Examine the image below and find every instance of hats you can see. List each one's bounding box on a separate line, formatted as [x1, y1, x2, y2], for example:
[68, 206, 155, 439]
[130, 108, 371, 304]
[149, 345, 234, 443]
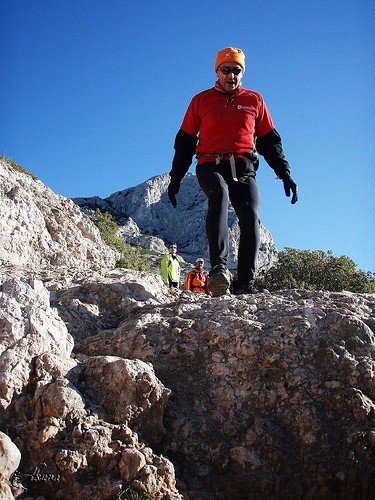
[196, 258, 204, 262]
[214, 47, 245, 73]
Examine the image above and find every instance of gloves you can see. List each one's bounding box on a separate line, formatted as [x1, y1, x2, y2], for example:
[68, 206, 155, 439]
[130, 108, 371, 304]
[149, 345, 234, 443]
[167, 176, 180, 207]
[281, 172, 298, 204]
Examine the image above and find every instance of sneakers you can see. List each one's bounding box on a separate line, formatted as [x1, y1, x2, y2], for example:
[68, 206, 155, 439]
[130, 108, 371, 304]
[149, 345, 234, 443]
[207, 265, 231, 296]
[238, 284, 271, 295]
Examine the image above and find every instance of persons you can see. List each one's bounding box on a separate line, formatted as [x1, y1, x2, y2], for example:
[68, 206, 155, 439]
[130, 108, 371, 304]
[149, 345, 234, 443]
[168, 47, 298, 297]
[161, 242, 180, 289]
[184, 258, 212, 298]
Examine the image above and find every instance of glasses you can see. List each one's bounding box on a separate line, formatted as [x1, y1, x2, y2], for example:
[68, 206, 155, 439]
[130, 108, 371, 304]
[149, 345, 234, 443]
[170, 247, 177, 248]
[218, 66, 242, 74]
